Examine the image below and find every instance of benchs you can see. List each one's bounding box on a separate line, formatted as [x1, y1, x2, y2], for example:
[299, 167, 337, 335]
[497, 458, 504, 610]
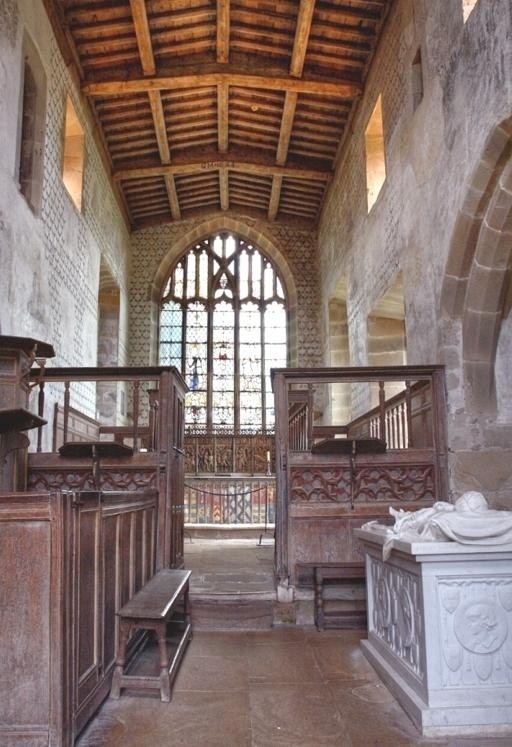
[296, 562, 368, 632]
[109, 567, 194, 703]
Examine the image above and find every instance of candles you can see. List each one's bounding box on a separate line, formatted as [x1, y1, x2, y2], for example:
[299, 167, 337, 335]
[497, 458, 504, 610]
[266, 451, 270, 463]
